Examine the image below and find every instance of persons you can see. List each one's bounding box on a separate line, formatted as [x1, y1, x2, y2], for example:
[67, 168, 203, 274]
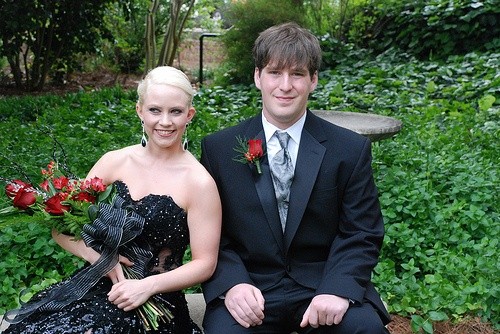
[0, 66, 222, 334]
[202, 22, 392, 334]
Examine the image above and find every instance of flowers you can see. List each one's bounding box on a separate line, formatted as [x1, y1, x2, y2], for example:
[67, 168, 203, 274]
[2, 158, 179, 333]
[231, 134, 268, 175]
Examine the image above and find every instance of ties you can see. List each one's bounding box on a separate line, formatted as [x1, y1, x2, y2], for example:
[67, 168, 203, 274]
[270, 131, 296, 232]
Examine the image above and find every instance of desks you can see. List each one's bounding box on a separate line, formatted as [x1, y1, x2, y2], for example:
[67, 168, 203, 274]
[307, 111, 402, 141]
